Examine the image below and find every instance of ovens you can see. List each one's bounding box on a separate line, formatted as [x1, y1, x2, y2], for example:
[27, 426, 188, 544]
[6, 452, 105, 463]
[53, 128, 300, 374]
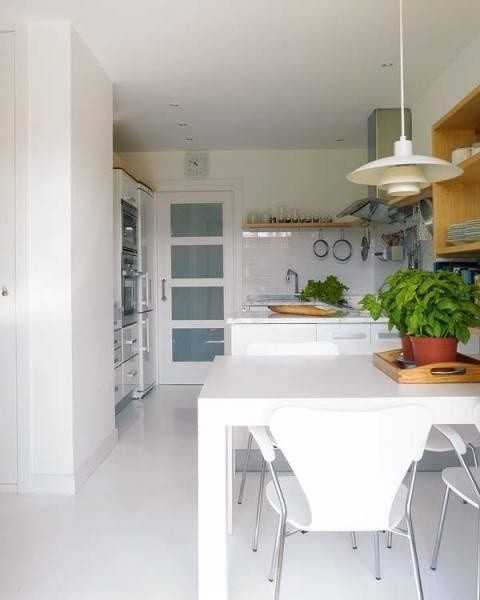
[119, 200, 141, 329]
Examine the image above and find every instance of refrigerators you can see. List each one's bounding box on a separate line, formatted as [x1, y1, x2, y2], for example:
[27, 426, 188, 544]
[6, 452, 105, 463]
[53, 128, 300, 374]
[136, 183, 156, 397]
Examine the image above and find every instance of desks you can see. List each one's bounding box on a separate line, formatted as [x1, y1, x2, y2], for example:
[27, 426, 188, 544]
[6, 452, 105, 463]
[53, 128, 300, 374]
[198, 354, 480, 600]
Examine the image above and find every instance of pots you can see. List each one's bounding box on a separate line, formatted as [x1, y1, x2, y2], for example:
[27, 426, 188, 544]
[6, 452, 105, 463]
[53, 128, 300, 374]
[313, 228, 329, 259]
[360, 228, 369, 261]
[333, 229, 352, 262]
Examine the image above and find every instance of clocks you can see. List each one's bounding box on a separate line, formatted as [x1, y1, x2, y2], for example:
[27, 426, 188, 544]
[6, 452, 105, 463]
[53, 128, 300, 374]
[183, 150, 210, 179]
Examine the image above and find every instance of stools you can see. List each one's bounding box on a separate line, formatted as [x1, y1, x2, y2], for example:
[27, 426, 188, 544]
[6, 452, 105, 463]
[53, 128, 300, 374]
[431, 405, 480, 568]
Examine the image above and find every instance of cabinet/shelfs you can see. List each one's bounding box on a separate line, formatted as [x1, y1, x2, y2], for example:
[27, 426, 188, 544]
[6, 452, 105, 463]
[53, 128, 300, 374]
[114, 322, 140, 414]
[429, 85, 479, 261]
[230, 324, 372, 353]
[362, 323, 402, 350]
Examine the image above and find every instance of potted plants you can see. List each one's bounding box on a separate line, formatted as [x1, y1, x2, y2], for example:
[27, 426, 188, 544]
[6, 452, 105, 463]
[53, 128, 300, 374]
[361, 266, 417, 361]
[398, 266, 479, 365]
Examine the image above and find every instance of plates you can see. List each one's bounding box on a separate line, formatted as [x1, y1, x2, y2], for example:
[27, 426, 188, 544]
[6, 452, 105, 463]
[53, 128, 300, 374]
[445, 219, 480, 245]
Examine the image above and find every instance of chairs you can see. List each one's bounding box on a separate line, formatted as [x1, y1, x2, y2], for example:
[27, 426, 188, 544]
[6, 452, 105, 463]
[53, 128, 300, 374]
[375, 336, 479, 547]
[242, 412, 434, 600]
[238, 341, 358, 552]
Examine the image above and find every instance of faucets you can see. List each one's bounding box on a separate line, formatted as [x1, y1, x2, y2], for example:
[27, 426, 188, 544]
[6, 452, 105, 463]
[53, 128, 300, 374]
[285, 268, 299, 294]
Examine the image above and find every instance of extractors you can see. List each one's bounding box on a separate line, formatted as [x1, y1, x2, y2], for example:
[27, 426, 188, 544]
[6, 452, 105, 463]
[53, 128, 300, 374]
[335, 109, 412, 223]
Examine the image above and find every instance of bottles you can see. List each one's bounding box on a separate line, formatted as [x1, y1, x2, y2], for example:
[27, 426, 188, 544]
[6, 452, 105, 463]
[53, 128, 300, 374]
[247, 206, 333, 224]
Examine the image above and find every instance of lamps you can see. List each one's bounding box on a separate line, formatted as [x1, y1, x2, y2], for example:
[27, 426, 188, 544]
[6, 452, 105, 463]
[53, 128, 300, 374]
[345, 1, 466, 197]
